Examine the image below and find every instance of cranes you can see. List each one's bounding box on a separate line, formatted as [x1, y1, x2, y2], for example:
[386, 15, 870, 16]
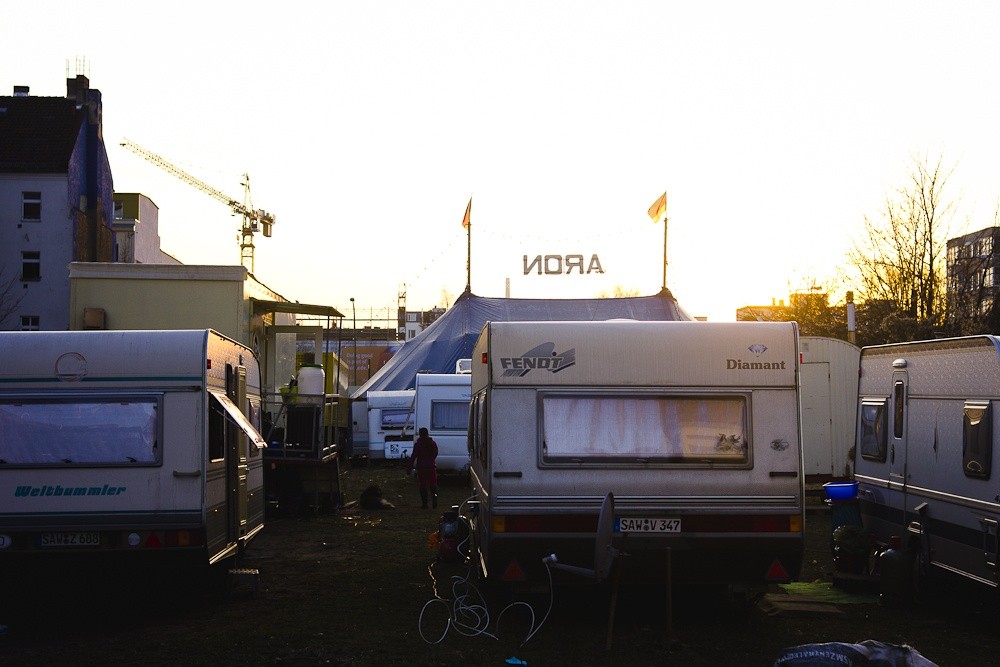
[118, 136, 276, 274]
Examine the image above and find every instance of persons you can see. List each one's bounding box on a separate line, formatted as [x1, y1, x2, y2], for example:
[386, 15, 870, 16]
[408, 428, 439, 509]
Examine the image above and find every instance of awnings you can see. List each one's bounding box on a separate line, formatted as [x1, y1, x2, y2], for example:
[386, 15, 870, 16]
[210, 390, 268, 448]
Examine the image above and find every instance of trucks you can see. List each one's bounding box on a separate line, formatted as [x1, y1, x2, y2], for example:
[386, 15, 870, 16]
[0, 329, 269, 606]
[798, 335, 861, 484]
[66, 261, 366, 515]
[415, 357, 472, 475]
[853, 331, 1000, 606]
[365, 388, 415, 465]
[466, 316, 807, 596]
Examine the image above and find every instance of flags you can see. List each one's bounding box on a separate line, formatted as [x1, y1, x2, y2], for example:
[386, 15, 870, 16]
[462, 200, 470, 229]
[647, 193, 667, 224]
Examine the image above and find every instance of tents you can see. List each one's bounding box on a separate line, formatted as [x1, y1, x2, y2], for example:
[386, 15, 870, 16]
[349, 292, 699, 399]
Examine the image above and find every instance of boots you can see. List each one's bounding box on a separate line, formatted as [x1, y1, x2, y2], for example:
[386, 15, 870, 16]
[431, 495, 438, 508]
[420, 490, 429, 509]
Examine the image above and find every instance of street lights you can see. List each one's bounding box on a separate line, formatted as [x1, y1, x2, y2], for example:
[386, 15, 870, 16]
[350, 298, 357, 386]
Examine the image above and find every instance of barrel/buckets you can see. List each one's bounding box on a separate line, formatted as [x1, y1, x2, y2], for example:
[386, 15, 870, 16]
[279, 386, 298, 405]
[298, 363, 325, 406]
